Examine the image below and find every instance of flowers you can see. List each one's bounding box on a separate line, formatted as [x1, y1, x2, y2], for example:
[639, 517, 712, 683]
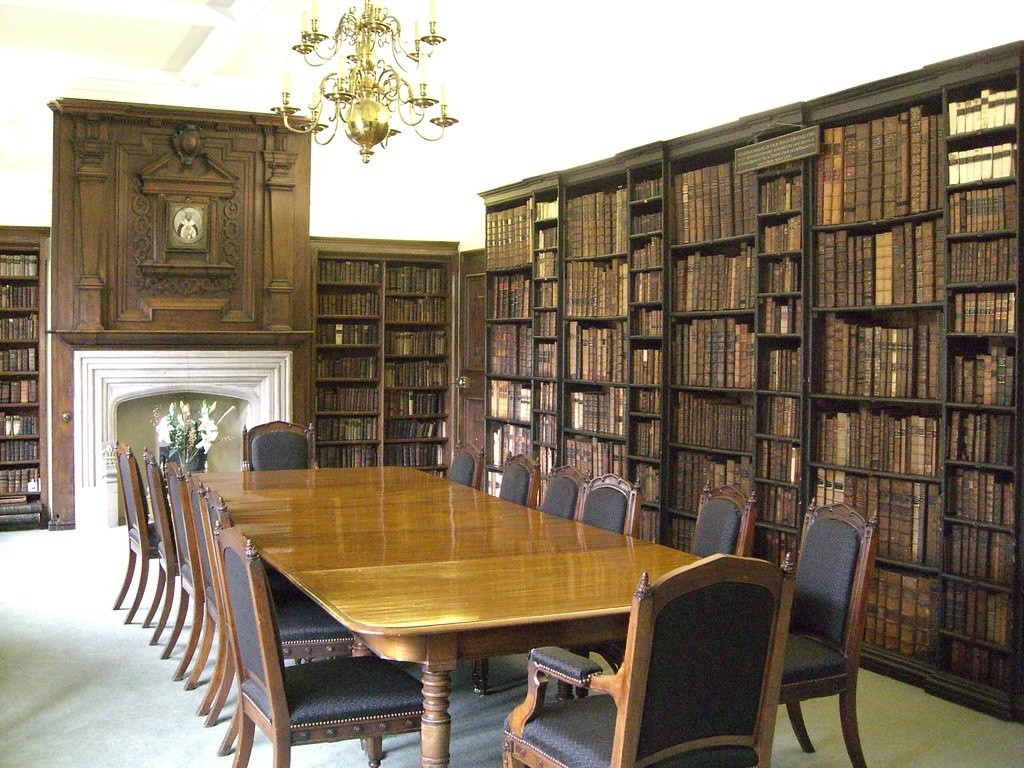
[151, 392, 236, 476]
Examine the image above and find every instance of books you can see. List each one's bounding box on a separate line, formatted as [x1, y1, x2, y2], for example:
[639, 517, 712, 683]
[300, 78, 1019, 711]
[1, 243, 57, 515]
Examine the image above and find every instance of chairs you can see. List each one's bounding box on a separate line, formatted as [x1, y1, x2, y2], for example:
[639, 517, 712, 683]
[198, 487, 352, 758]
[571, 468, 644, 537]
[688, 475, 758, 566]
[184, 471, 229, 717]
[535, 459, 583, 527]
[448, 441, 486, 494]
[108, 438, 176, 626]
[156, 458, 209, 681]
[241, 422, 319, 474]
[496, 551, 796, 767]
[209, 517, 452, 767]
[772, 495, 880, 768]
[141, 446, 191, 646]
[497, 449, 540, 511]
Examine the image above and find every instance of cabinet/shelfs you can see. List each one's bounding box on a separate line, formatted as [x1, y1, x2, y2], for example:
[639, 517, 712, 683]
[308, 236, 459, 484]
[482, 40, 1023, 725]
[0, 223, 53, 530]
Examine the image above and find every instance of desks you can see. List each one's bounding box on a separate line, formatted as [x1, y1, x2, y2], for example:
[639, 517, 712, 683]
[187, 461, 717, 766]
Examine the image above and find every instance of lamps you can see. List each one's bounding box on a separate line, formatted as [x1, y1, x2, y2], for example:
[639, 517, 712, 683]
[273, 0, 460, 164]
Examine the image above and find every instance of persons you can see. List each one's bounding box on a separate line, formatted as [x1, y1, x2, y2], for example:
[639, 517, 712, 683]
[177, 211, 199, 239]
